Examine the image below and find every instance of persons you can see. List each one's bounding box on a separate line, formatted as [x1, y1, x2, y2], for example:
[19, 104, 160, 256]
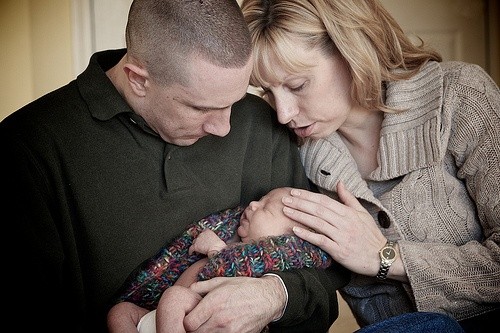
[109, 187, 311, 333]
[240, 0, 500, 333]
[0, 0, 339, 333]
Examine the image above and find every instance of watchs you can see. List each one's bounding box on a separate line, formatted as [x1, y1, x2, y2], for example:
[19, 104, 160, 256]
[376, 239, 398, 280]
